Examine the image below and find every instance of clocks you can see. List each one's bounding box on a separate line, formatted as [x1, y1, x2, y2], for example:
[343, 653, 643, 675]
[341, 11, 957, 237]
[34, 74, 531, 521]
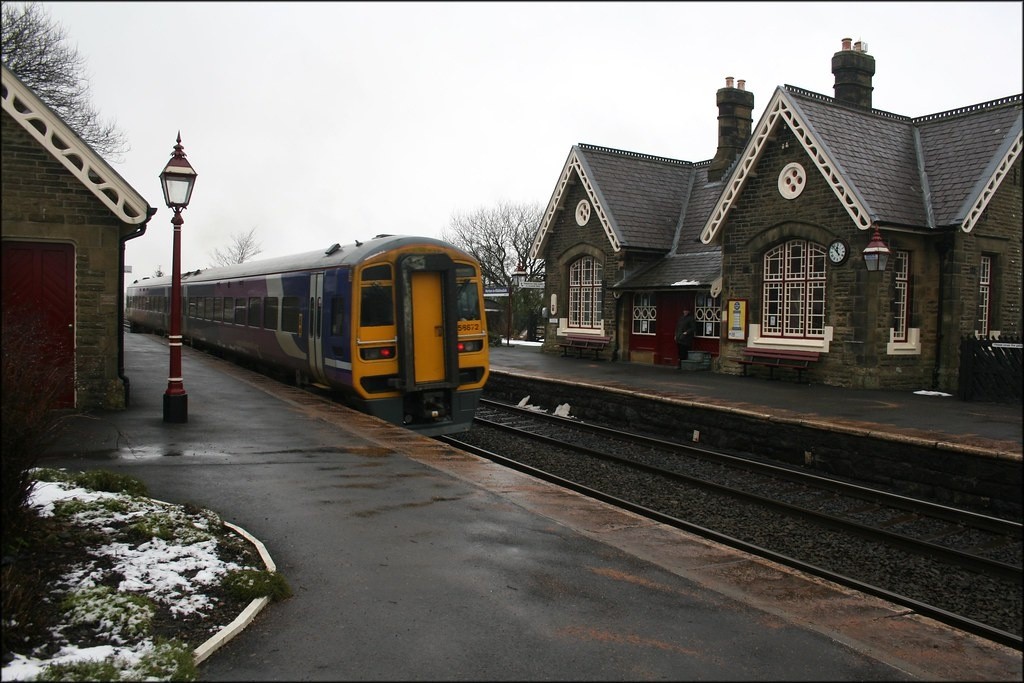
[828, 238, 849, 265]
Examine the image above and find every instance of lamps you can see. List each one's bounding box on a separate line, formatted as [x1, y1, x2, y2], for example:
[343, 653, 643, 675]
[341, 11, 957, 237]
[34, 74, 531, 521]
[863, 223, 892, 271]
[511, 263, 547, 286]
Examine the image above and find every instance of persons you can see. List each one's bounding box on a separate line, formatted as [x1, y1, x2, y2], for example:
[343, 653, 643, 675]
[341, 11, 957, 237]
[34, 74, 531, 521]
[673, 306, 697, 370]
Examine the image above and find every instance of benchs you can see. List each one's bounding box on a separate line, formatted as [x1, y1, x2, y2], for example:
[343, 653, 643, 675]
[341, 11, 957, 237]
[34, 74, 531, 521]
[734, 347, 820, 382]
[559, 333, 611, 360]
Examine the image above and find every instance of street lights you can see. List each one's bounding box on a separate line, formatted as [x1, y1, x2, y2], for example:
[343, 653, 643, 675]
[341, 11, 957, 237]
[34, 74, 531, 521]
[161, 129, 198, 426]
[506, 284, 514, 347]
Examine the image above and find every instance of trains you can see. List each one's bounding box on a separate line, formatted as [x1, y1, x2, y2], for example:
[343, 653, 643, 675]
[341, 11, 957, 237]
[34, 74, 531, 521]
[124, 232, 489, 437]
